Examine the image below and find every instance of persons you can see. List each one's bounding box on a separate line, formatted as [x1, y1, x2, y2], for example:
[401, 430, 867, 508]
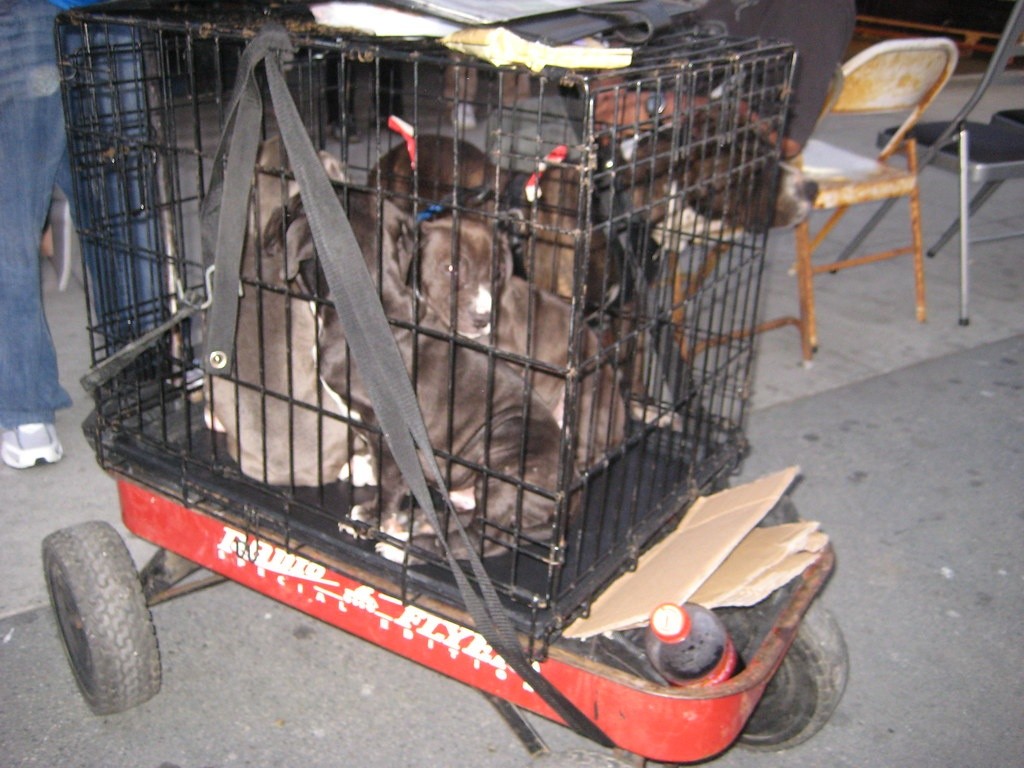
[1, 0, 202, 469]
[319, 39, 531, 142]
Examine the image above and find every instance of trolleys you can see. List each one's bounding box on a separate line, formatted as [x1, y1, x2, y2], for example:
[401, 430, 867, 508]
[34, 0, 855, 768]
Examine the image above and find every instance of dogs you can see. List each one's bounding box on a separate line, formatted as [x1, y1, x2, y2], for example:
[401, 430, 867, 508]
[202, 103, 818, 572]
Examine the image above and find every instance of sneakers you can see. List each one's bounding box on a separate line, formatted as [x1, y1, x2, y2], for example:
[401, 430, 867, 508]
[0, 422, 62, 469]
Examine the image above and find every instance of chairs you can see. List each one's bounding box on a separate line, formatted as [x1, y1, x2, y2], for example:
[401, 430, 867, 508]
[670, 38, 960, 369]
[826, 0, 1024, 327]
